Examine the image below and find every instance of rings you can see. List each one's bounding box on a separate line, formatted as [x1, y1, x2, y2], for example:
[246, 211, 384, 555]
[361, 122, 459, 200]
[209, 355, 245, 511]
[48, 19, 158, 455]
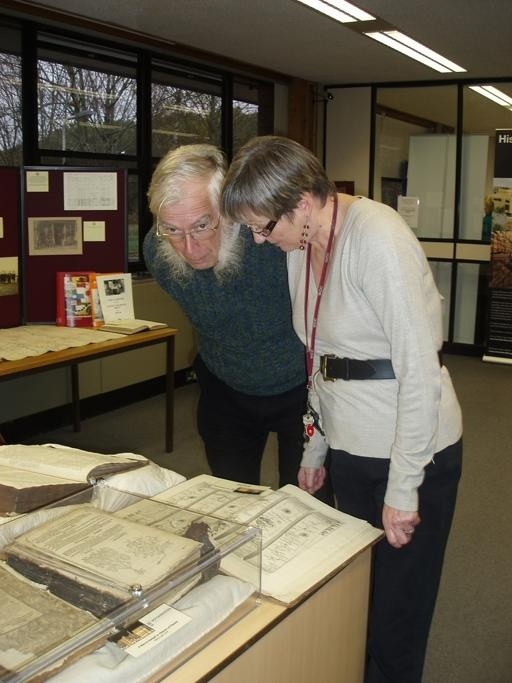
[403, 526, 415, 534]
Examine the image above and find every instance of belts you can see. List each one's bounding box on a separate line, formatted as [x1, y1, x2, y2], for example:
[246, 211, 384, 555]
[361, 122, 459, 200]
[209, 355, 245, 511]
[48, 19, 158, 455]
[320, 349, 443, 382]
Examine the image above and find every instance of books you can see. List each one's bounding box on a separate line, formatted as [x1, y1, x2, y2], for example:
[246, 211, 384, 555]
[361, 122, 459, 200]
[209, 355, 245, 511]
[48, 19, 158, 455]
[97, 317, 170, 335]
[88, 270, 125, 326]
[1, 443, 385, 681]
[96, 271, 136, 323]
[55, 270, 112, 328]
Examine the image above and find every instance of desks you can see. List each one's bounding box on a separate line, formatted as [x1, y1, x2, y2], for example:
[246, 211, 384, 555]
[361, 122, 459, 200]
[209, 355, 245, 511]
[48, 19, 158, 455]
[0, 440, 388, 681]
[1, 314, 179, 454]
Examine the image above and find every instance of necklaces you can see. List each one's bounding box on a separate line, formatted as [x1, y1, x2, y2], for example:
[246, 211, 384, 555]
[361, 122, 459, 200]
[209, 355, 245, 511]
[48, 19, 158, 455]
[299, 189, 338, 440]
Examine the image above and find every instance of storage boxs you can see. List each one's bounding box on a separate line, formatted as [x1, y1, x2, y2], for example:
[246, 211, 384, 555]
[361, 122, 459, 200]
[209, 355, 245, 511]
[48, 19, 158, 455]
[0, 482, 262, 681]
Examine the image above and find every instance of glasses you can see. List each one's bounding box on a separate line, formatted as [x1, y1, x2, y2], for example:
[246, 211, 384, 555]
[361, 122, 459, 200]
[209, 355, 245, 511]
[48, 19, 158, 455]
[156, 217, 220, 241]
[246, 215, 280, 238]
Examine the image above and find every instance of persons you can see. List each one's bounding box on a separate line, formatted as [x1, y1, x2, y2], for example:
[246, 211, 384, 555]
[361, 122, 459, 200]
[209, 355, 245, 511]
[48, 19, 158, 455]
[144, 141, 329, 499]
[221, 133, 464, 676]
